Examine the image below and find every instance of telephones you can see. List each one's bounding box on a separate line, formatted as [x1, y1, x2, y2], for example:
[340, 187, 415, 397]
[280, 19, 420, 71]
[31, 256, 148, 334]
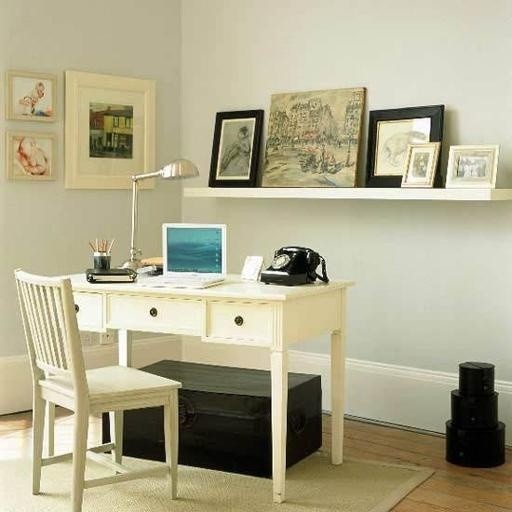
[261, 246, 330, 284]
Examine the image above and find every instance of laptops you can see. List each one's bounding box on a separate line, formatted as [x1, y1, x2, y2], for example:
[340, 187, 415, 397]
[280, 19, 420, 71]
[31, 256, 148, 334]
[137, 222, 229, 290]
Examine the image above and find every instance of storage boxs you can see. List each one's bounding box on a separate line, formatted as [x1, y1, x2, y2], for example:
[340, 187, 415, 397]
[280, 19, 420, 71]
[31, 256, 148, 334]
[100, 358, 320, 479]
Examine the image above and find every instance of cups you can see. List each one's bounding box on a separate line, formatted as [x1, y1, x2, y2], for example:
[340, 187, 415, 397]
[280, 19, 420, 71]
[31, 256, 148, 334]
[93, 251, 112, 270]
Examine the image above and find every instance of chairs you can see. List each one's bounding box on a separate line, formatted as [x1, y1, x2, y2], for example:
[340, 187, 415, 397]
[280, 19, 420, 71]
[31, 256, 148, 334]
[11, 267, 185, 512]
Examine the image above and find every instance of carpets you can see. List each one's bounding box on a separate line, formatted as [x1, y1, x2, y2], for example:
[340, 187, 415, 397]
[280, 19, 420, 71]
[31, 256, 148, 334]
[1, 435, 431, 512]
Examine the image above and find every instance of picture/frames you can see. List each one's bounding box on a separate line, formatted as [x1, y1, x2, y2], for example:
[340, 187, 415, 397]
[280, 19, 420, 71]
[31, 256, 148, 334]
[445, 143, 500, 188]
[401, 142, 441, 187]
[65, 70, 156, 191]
[6, 132, 58, 182]
[6, 70, 56, 122]
[208, 109, 263, 186]
[364, 105, 444, 187]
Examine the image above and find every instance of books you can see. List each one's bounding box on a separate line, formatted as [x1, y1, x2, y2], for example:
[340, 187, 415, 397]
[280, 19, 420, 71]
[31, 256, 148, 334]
[86, 268, 137, 283]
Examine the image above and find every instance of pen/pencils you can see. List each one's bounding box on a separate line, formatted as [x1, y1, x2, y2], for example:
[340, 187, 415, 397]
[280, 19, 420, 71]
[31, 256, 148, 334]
[87, 238, 116, 254]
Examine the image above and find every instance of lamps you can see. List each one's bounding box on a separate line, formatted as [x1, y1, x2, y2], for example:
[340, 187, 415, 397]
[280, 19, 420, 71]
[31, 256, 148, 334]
[119, 159, 200, 267]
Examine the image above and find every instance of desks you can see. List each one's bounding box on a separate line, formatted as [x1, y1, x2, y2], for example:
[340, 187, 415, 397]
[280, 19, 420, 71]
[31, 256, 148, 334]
[43, 273, 348, 503]
